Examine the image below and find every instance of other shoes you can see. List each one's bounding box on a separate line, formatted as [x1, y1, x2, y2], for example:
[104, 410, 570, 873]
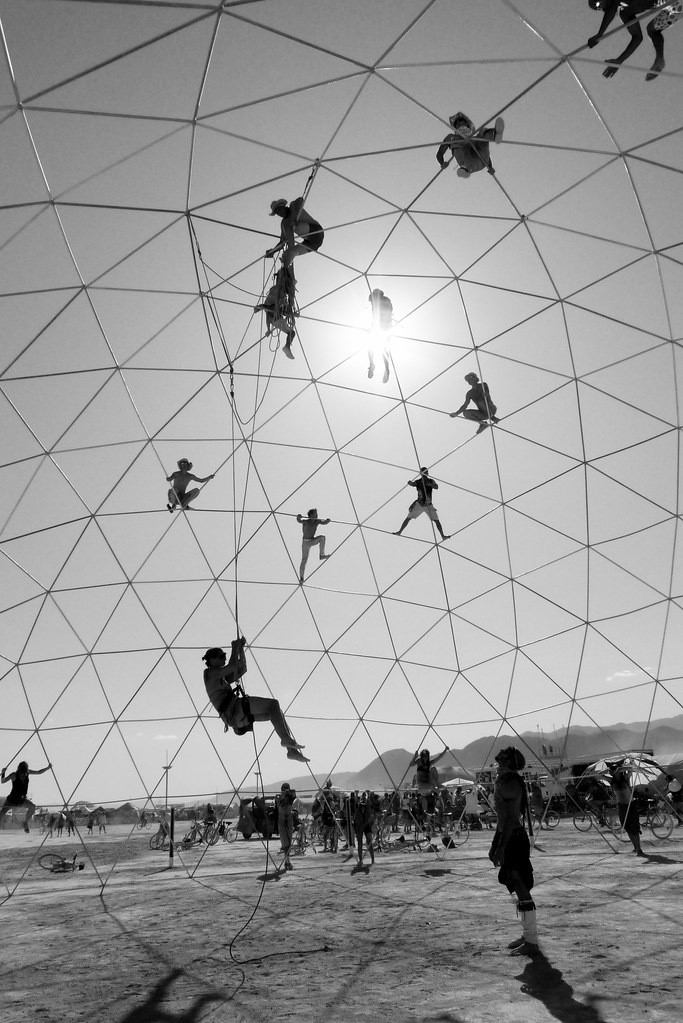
[22, 822, 30, 834]
[281, 739, 311, 763]
[382, 369, 390, 384]
[368, 364, 375, 378]
[393, 531, 401, 535]
[320, 555, 330, 560]
[282, 347, 295, 359]
[171, 504, 194, 509]
[632, 848, 644, 855]
[508, 936, 539, 956]
[495, 117, 504, 144]
[476, 418, 501, 434]
[284, 862, 294, 870]
[300, 578, 304, 583]
[442, 535, 450, 540]
[457, 167, 470, 179]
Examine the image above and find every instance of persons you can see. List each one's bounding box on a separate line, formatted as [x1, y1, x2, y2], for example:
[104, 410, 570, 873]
[449, 370, 497, 434]
[586, 0, 683, 82]
[291, 773, 555, 854]
[391, 466, 451, 540]
[201, 635, 310, 762]
[165, 456, 215, 513]
[265, 197, 325, 268]
[296, 507, 330, 584]
[410, 746, 452, 816]
[218, 820, 226, 842]
[139, 810, 147, 828]
[264, 266, 297, 359]
[488, 745, 539, 957]
[367, 287, 393, 384]
[38, 810, 107, 837]
[435, 110, 504, 178]
[354, 789, 376, 869]
[0, 761, 53, 833]
[597, 763, 644, 856]
[202, 803, 217, 828]
[275, 782, 296, 871]
[155, 815, 169, 848]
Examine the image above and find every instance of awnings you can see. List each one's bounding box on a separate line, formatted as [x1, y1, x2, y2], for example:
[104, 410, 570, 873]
[440, 777, 487, 806]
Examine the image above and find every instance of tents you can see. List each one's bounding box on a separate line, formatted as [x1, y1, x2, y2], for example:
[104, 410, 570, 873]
[292, 797, 316, 813]
[312, 785, 350, 801]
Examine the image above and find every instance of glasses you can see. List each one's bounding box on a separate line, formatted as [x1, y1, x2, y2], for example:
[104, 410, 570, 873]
[512, 746, 520, 768]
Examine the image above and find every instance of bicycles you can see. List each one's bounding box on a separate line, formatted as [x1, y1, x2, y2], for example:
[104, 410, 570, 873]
[36, 789, 683, 874]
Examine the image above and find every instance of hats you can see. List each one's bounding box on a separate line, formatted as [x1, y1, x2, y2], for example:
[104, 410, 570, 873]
[268, 199, 288, 216]
[177, 458, 194, 471]
[449, 112, 474, 131]
[465, 372, 479, 382]
[369, 287, 384, 301]
